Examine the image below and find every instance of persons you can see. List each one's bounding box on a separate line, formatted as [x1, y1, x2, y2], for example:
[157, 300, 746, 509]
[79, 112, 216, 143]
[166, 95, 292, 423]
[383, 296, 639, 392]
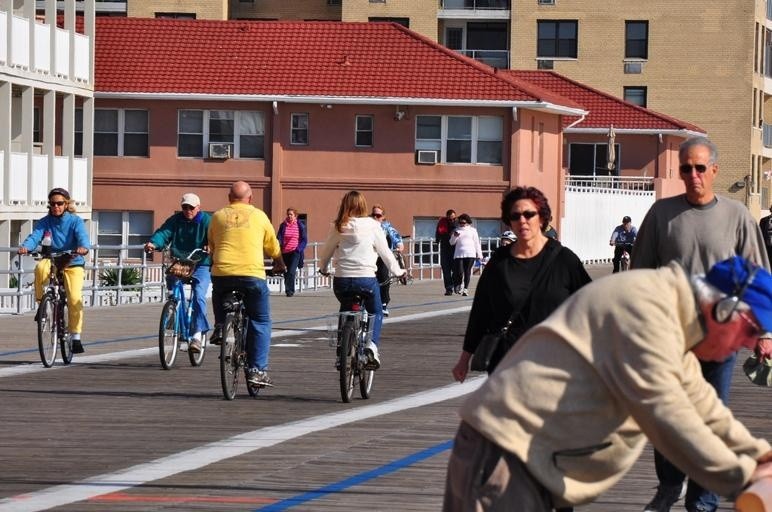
[208, 181, 282, 386]
[372, 204, 405, 314]
[452, 187, 592, 384]
[632, 138, 770, 511]
[759, 206, 772, 268]
[442, 258, 771, 512]
[320, 190, 407, 371]
[450, 214, 483, 296]
[435, 209, 463, 295]
[501, 231, 517, 247]
[276, 208, 308, 297]
[144, 193, 212, 353]
[545, 216, 558, 241]
[610, 216, 638, 274]
[18, 188, 89, 353]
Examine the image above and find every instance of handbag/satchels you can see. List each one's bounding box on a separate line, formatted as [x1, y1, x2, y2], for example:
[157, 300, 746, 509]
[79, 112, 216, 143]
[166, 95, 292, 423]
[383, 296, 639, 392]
[470, 333, 501, 372]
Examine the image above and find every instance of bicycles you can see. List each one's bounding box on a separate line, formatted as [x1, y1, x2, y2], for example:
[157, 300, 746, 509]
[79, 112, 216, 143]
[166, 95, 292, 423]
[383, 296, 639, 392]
[610, 240, 635, 274]
[145, 243, 215, 370]
[11, 245, 90, 367]
[394, 233, 417, 284]
[214, 282, 268, 399]
[315, 265, 413, 405]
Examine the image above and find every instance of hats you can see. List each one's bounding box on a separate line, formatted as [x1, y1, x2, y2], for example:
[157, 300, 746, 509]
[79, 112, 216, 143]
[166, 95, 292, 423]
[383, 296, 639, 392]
[704, 256, 771, 333]
[180, 193, 201, 208]
[49, 188, 69, 199]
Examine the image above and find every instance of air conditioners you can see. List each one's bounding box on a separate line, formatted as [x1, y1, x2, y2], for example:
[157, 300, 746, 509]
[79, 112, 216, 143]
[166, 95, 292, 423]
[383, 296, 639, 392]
[418, 150, 437, 165]
[210, 144, 230, 159]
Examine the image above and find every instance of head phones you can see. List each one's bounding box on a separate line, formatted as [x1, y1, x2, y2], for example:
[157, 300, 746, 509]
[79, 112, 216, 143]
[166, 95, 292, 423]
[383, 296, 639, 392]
[713, 265, 761, 323]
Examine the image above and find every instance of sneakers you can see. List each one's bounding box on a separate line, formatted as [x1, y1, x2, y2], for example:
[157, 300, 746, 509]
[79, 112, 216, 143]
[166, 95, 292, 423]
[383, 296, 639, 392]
[210, 323, 224, 343]
[71, 339, 84, 353]
[364, 338, 381, 369]
[188, 338, 201, 352]
[643, 480, 688, 512]
[247, 367, 276, 387]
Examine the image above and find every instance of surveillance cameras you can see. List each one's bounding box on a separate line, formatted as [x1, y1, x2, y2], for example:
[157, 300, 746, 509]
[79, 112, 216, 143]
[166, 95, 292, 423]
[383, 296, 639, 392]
[393, 111, 404, 121]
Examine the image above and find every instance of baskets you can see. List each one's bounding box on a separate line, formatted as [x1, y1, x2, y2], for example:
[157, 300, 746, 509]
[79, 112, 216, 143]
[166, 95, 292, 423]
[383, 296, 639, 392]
[163, 254, 202, 279]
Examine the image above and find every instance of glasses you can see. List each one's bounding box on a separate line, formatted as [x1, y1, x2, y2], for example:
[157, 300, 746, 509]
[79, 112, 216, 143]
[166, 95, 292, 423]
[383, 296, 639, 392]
[508, 210, 539, 222]
[680, 162, 710, 174]
[48, 200, 66, 206]
[371, 212, 382, 218]
[181, 206, 195, 210]
